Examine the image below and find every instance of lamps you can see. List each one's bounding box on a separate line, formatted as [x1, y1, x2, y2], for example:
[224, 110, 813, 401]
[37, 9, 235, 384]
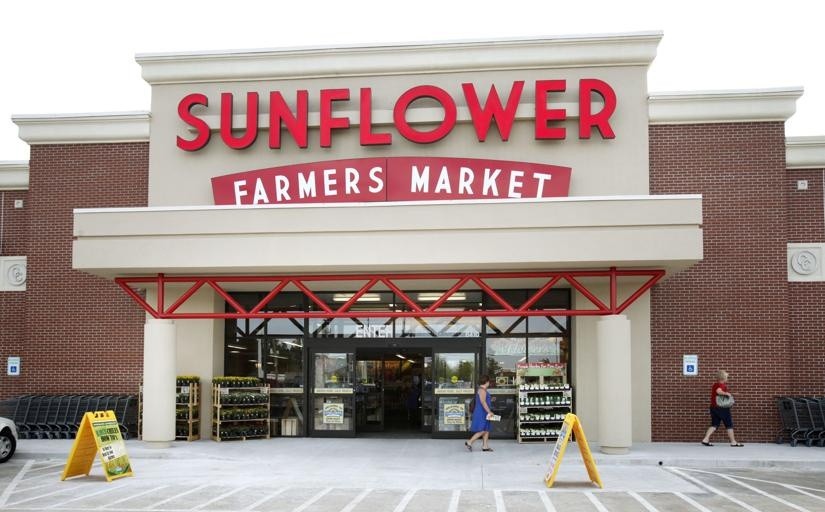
[333, 294, 381, 302]
[417, 293, 466, 301]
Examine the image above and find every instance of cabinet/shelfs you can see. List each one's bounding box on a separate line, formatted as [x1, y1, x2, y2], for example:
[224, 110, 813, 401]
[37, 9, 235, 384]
[138, 381, 202, 442]
[515, 363, 573, 444]
[211, 383, 270, 442]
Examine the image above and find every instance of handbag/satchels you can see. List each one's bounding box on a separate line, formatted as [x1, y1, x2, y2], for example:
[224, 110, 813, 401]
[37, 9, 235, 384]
[716, 395, 734, 408]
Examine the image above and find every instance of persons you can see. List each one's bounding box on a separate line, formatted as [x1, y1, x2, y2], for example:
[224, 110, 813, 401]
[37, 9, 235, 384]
[405, 378, 420, 425]
[463, 374, 495, 453]
[700, 369, 745, 448]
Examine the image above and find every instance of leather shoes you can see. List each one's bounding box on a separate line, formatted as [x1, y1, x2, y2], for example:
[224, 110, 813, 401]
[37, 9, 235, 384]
[730, 443, 745, 447]
[701, 441, 715, 446]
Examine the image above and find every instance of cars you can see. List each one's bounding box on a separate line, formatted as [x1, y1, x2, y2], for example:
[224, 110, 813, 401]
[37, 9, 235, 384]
[0, 418, 18, 463]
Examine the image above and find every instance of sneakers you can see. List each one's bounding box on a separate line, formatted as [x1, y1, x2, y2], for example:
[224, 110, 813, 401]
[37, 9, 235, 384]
[465, 441, 472, 452]
[483, 447, 493, 451]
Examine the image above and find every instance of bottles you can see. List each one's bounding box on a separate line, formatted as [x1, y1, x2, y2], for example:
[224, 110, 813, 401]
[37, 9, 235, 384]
[174, 376, 200, 436]
[211, 376, 267, 420]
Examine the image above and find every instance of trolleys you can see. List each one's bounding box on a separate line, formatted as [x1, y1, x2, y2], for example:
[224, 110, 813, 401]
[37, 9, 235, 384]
[0, 393, 138, 440]
[775, 395, 824, 446]
